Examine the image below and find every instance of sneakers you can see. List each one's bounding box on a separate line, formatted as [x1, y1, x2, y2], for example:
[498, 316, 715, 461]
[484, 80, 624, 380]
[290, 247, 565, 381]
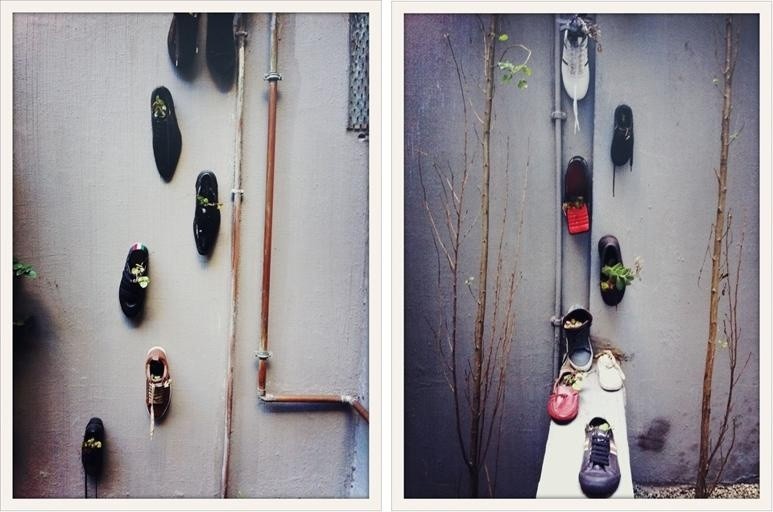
[610, 104, 634, 197]
[145, 346, 172, 440]
[560, 17, 590, 133]
[82, 417, 105, 498]
[563, 156, 589, 235]
[594, 349, 625, 392]
[578, 416, 621, 498]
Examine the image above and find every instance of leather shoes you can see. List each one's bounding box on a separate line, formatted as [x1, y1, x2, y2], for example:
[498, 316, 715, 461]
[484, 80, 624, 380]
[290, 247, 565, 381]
[193, 169, 221, 256]
[598, 235, 626, 311]
[167, 12, 201, 83]
[561, 304, 593, 373]
[119, 243, 150, 319]
[150, 85, 183, 183]
[547, 371, 579, 424]
[205, 14, 237, 95]
